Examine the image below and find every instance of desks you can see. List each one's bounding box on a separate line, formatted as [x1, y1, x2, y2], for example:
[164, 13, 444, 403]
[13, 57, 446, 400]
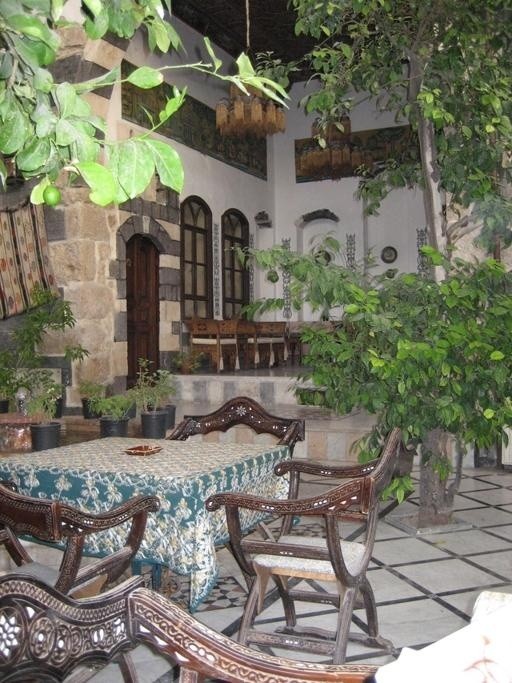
[1, 432, 293, 605]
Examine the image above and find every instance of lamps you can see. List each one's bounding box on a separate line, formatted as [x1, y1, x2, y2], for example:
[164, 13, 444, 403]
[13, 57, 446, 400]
[214, 0, 288, 139]
[296, 32, 371, 182]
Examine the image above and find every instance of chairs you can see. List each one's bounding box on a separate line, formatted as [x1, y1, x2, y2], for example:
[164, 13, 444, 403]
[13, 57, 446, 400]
[181, 313, 355, 371]
[0, 482, 163, 601]
[126, 589, 390, 681]
[206, 419, 403, 667]
[164, 394, 307, 459]
[0, 572, 150, 683]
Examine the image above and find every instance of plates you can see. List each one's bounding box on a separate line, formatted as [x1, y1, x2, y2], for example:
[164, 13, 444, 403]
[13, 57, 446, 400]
[122, 445, 163, 456]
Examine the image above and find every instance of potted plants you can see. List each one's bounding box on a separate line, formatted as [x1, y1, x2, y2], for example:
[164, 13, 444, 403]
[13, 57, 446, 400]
[1, 278, 180, 453]
